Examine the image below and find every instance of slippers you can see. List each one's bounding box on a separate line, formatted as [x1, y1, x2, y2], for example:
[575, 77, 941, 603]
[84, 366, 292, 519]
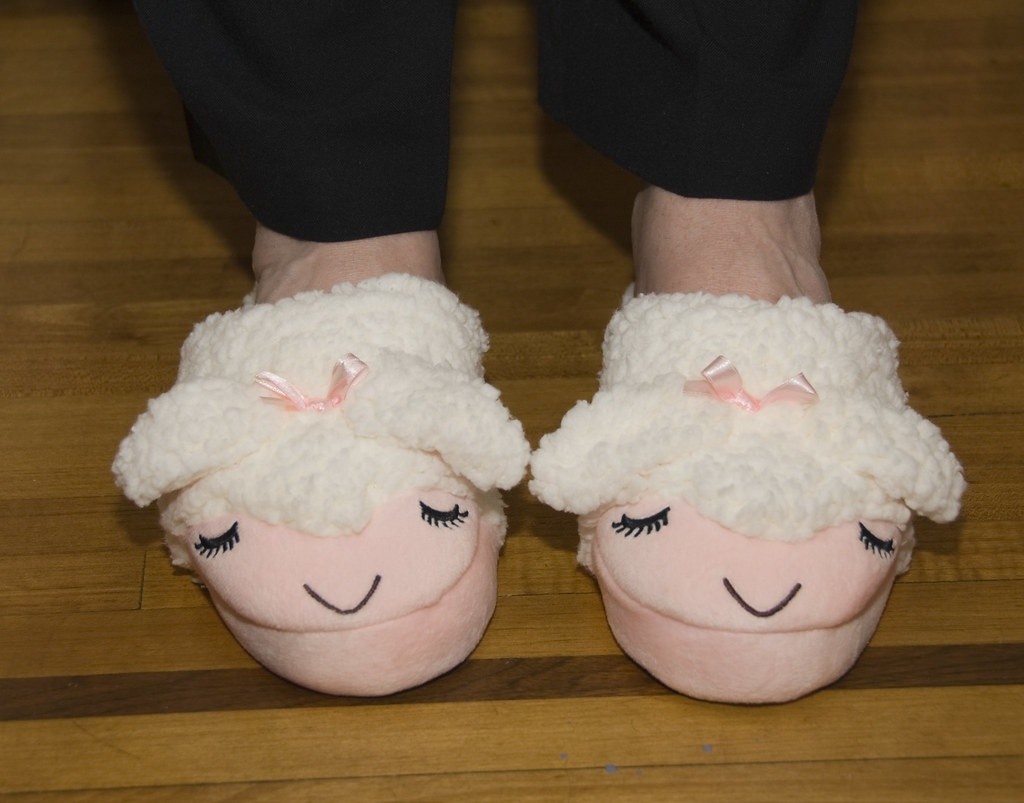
[530, 284, 967, 707]
[111, 273, 531, 696]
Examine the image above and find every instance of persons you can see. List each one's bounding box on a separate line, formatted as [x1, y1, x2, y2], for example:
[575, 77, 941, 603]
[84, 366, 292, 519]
[111, 0, 967, 704]
